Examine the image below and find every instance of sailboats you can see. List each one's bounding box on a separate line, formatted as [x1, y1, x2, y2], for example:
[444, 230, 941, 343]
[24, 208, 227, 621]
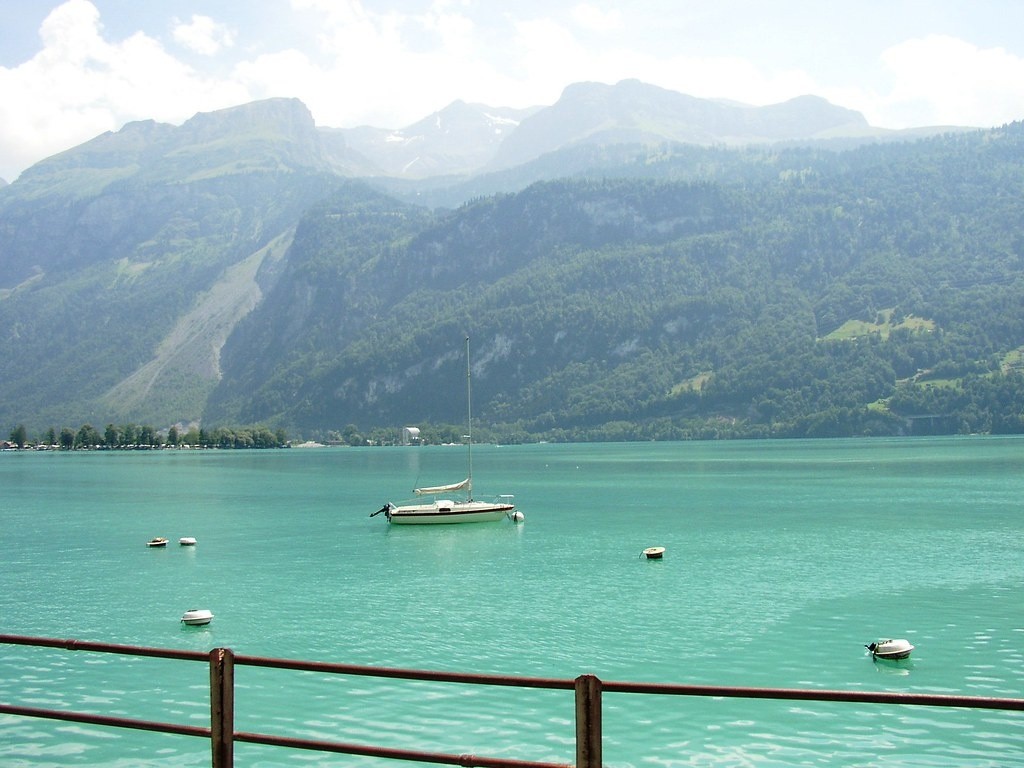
[365, 333, 518, 526]
